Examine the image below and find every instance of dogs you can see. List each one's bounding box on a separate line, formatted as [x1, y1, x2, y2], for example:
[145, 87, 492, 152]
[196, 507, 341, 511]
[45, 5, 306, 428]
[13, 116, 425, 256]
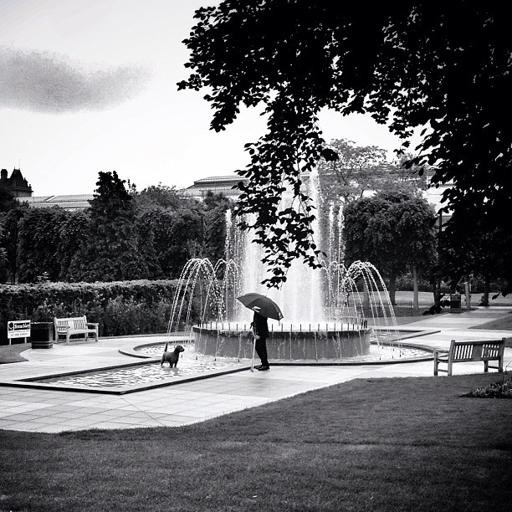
[160, 343, 184, 368]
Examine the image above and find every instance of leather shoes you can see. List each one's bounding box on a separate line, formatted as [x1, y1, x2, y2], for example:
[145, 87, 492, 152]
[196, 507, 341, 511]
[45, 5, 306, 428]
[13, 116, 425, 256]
[254, 365, 269, 371]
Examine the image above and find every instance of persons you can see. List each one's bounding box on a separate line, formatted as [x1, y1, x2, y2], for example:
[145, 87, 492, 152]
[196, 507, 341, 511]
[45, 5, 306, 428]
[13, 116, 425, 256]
[250, 310, 270, 371]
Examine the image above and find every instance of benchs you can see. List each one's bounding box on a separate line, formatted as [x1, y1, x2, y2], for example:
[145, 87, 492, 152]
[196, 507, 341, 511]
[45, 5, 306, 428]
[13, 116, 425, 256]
[54, 315, 100, 344]
[433, 337, 507, 376]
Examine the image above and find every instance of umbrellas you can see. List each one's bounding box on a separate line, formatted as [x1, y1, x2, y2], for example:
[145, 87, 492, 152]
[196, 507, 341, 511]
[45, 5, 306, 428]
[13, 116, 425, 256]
[236, 293, 284, 329]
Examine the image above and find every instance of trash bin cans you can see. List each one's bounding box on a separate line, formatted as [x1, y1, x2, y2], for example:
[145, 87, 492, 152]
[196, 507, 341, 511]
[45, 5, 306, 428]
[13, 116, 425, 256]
[451, 294, 461, 313]
[30, 322, 54, 349]
